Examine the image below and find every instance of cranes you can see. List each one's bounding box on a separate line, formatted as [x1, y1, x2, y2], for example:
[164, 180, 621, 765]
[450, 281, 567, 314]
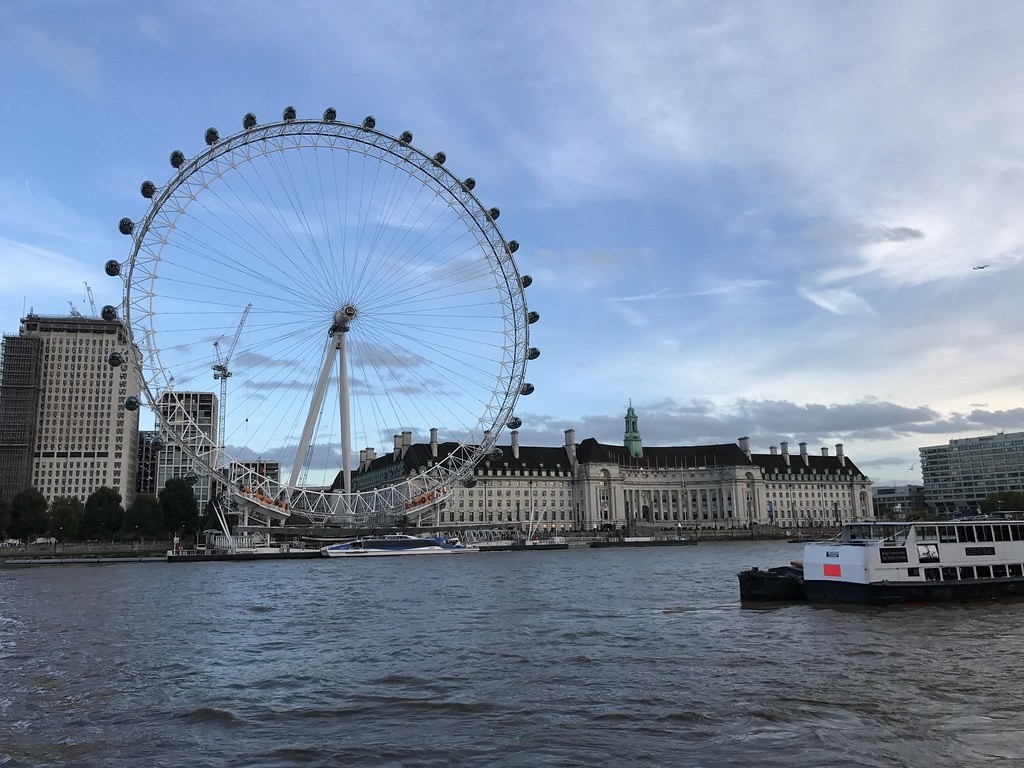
[211, 301, 254, 499]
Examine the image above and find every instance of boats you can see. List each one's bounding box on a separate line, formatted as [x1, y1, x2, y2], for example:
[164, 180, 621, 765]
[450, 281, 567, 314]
[802, 513, 1024, 602]
[736, 562, 805, 610]
[319, 535, 481, 558]
